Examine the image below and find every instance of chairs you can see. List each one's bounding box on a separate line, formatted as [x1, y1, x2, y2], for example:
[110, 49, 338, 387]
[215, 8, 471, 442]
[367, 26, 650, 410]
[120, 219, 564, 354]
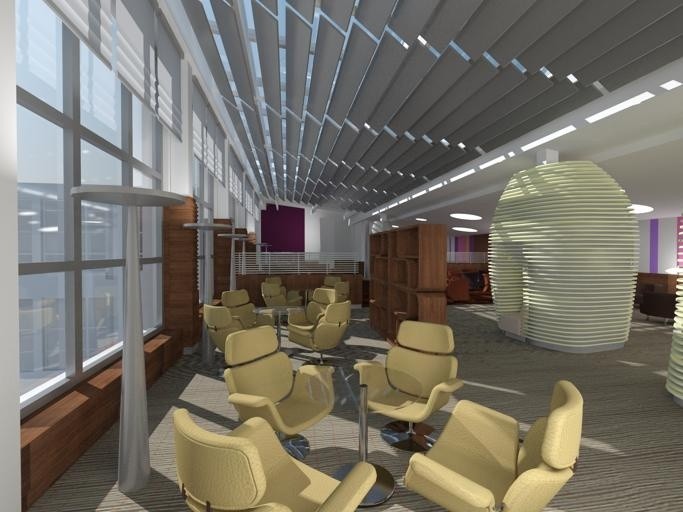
[173, 408, 378, 510]
[354, 320, 464, 452]
[405, 380, 583, 510]
[288, 301, 352, 365]
[221, 276, 352, 355]
[203, 303, 246, 377]
[222, 326, 334, 461]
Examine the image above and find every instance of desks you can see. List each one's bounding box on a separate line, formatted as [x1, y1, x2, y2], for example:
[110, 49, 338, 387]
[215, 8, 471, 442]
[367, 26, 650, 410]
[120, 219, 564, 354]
[306, 365, 423, 508]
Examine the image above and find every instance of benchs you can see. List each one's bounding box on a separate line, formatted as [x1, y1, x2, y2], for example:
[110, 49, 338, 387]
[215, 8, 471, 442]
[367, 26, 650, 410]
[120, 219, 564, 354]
[637, 290, 676, 326]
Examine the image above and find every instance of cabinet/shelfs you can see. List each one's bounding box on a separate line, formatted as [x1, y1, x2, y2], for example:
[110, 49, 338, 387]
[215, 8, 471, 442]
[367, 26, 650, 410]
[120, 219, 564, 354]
[387, 224, 450, 346]
[368, 231, 388, 338]
